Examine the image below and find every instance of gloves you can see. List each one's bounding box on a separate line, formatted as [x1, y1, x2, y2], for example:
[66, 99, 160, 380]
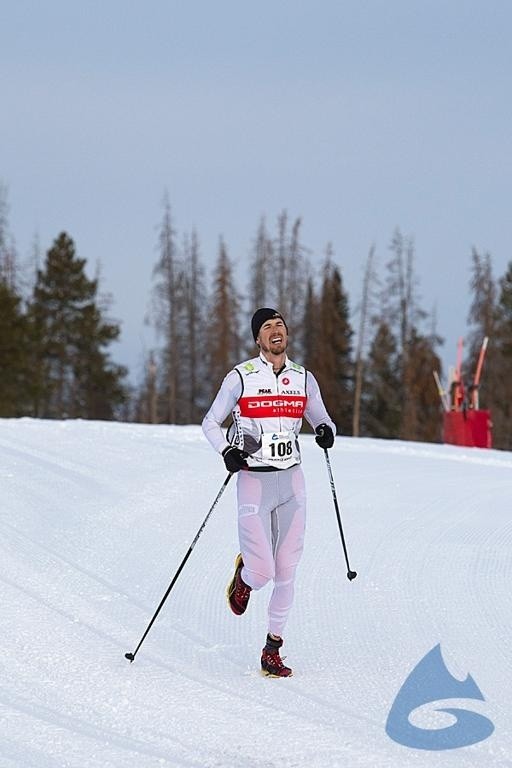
[222, 446, 250, 473]
[316, 422, 334, 449]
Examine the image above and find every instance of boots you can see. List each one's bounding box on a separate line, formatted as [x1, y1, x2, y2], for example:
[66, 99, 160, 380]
[227, 553, 252, 616]
[261, 632, 291, 679]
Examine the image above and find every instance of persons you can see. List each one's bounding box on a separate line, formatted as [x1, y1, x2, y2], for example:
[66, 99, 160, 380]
[201, 305, 337, 679]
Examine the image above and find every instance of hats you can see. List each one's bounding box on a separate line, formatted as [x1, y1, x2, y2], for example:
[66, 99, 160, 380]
[251, 307, 289, 343]
